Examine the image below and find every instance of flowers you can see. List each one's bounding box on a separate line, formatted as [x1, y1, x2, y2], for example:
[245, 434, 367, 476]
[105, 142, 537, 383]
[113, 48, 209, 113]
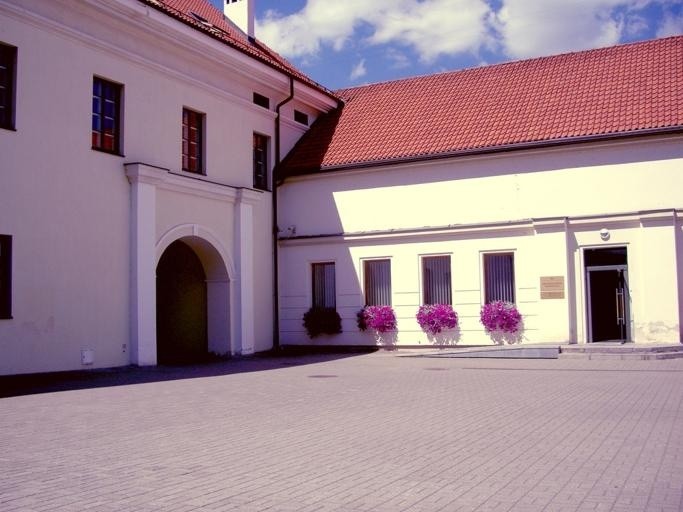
[355, 305, 398, 331]
[415, 303, 457, 335]
[303, 305, 342, 338]
[478, 300, 522, 333]
